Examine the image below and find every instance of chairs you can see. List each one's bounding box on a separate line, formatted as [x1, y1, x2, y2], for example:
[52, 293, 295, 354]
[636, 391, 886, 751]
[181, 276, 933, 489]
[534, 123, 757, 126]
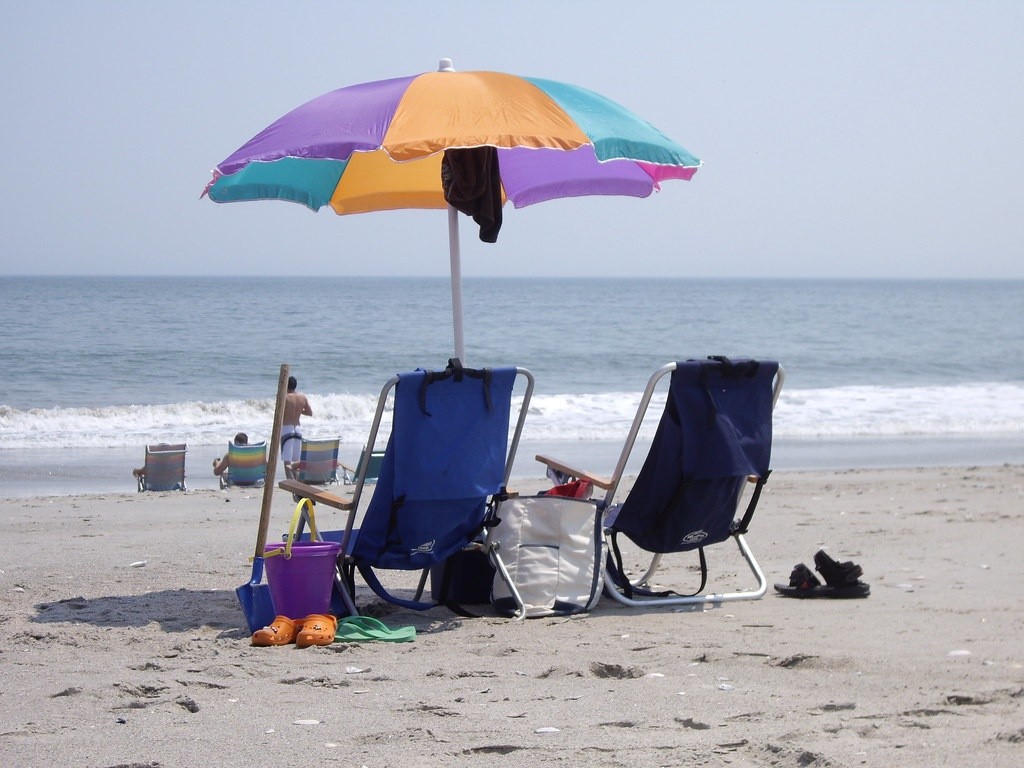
[286, 436, 341, 486]
[535, 353, 785, 608]
[278, 356, 535, 636]
[216, 440, 268, 490]
[338, 443, 385, 485]
[138, 443, 187, 492]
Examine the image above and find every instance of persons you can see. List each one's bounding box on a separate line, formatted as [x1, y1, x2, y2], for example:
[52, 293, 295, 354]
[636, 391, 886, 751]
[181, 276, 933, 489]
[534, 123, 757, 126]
[132, 442, 180, 489]
[213, 432, 248, 483]
[281, 376, 312, 480]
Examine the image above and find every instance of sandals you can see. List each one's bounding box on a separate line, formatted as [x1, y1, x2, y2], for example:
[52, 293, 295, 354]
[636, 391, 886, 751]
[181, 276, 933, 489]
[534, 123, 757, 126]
[773, 549, 874, 598]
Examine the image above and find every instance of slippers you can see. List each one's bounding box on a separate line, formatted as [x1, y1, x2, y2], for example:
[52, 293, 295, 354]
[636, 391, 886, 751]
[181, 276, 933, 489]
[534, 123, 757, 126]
[295, 612, 338, 647]
[251, 615, 306, 645]
[334, 615, 418, 643]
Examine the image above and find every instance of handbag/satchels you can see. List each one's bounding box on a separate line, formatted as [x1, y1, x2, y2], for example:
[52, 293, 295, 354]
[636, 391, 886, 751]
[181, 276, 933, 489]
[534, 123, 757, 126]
[490, 493, 613, 618]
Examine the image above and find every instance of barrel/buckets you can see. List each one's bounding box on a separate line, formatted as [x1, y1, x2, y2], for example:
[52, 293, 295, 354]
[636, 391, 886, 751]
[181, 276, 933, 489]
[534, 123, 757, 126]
[235, 498, 342, 635]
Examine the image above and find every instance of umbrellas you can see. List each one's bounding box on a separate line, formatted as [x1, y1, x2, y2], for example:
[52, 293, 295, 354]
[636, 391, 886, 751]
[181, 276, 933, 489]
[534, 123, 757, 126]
[196, 59, 707, 370]
